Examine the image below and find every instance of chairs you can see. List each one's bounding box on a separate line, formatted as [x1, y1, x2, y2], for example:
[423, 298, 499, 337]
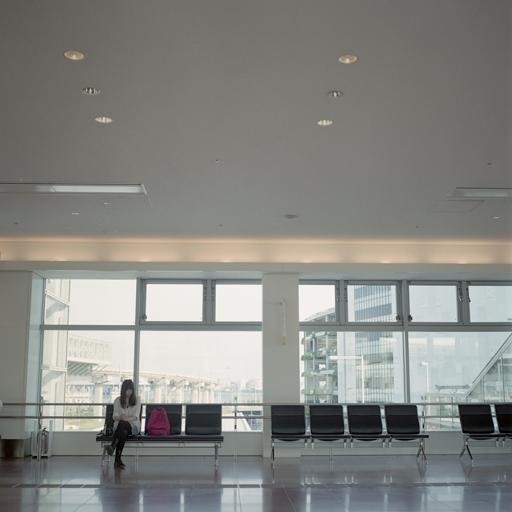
[457, 402, 511, 460]
[96, 403, 224, 462]
[270, 404, 429, 462]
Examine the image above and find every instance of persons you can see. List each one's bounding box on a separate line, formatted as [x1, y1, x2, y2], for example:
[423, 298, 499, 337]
[104, 381, 141, 469]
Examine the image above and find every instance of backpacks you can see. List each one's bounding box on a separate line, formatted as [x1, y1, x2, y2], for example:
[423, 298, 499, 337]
[146, 407, 171, 436]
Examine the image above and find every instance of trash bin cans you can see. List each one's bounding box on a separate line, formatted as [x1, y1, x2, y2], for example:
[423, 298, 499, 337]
[31, 430, 52, 458]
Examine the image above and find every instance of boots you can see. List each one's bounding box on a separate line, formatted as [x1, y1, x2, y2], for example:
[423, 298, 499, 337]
[114, 449, 126, 469]
[104, 437, 119, 456]
